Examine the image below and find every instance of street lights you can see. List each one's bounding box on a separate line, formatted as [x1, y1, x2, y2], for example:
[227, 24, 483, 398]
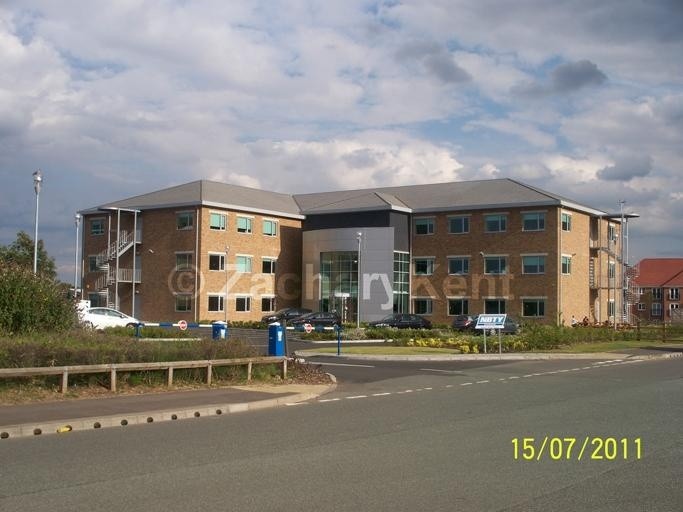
[357, 232, 362, 328]
[75, 213, 81, 298]
[225, 246, 230, 323]
[33, 170, 42, 273]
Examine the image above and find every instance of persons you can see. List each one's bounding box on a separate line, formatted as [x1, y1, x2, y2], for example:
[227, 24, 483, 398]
[571, 315, 577, 328]
[583, 316, 589, 326]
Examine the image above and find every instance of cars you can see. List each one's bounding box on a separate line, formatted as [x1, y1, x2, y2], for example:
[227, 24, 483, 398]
[77, 306, 140, 329]
[288, 310, 340, 326]
[369, 313, 432, 329]
[454, 314, 519, 335]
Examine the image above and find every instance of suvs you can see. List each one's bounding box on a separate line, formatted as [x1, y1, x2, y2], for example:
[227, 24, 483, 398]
[263, 306, 311, 323]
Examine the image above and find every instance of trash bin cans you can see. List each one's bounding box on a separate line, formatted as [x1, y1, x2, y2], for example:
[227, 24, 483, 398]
[211, 321, 227, 341]
[267, 322, 284, 355]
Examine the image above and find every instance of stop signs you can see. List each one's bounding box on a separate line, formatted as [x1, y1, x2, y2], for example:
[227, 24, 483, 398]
[179, 320, 188, 330]
[304, 324, 312, 333]
